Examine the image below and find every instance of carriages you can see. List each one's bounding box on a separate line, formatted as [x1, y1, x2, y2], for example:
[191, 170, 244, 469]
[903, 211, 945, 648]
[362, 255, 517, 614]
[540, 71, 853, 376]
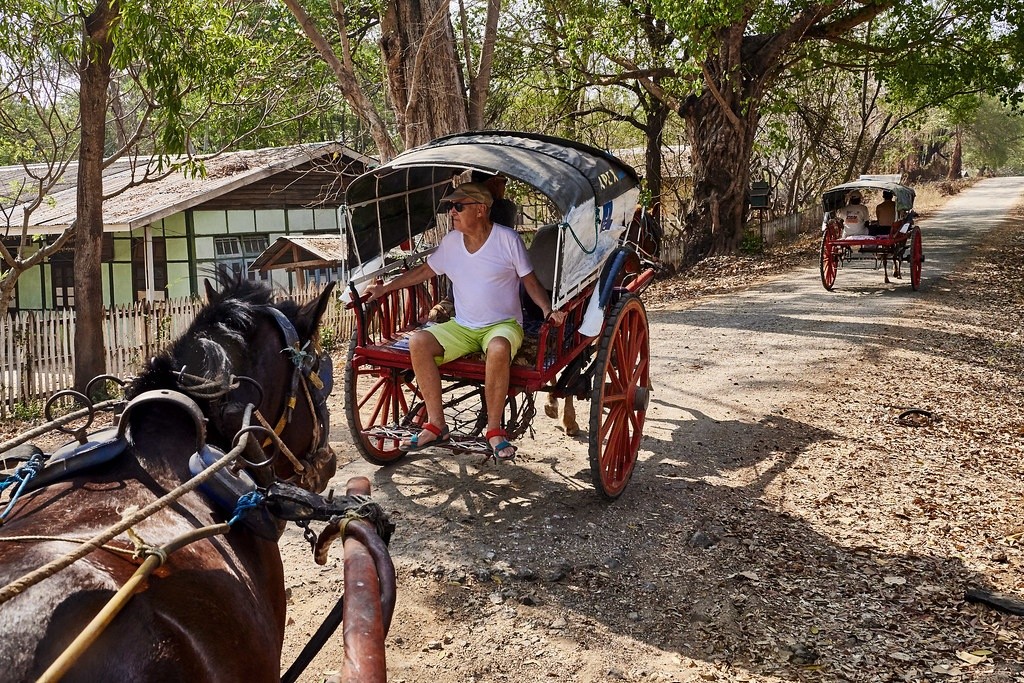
[814, 166, 928, 291]
[337, 128, 663, 506]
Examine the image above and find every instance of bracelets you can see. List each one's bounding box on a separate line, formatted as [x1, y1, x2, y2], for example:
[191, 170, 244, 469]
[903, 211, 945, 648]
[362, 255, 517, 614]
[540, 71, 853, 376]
[546, 310, 553, 320]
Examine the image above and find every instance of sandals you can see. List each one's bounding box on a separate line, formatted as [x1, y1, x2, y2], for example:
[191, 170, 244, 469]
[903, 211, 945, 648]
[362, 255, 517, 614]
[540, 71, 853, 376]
[400, 420, 450, 455]
[484, 428, 516, 460]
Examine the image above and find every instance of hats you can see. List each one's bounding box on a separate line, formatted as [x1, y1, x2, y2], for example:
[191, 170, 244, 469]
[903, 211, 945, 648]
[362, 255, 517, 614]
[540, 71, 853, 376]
[852, 190, 861, 199]
[440, 182, 494, 207]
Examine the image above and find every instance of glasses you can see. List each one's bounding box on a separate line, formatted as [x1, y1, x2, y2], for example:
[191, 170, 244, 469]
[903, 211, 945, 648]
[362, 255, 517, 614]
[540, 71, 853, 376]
[445, 200, 482, 211]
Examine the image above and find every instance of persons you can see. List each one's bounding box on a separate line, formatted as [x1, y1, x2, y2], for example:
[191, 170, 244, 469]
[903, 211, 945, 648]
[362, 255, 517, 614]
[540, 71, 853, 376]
[835, 190, 870, 239]
[366, 185, 564, 460]
[876, 190, 895, 235]
[523, 222, 560, 317]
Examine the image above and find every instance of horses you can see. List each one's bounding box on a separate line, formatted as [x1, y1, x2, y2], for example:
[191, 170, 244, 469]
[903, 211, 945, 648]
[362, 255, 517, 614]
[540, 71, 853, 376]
[520, 201, 663, 436]
[0, 258, 338, 683]
[881, 208, 921, 284]
